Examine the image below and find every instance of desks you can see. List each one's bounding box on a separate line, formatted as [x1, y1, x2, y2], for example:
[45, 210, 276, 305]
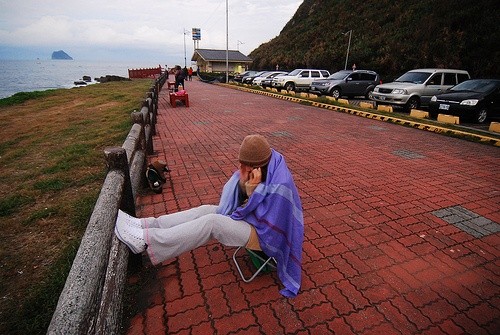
[170, 92, 189, 108]
[167, 81, 176, 90]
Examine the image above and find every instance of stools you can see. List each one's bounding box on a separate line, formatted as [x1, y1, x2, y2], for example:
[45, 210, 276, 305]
[232, 246, 278, 283]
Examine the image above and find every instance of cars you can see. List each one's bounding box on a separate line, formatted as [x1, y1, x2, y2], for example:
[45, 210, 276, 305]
[228, 70, 290, 90]
[428, 78, 500, 124]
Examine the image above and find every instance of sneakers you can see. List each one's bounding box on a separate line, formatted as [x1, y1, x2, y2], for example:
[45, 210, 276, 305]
[113, 209, 148, 255]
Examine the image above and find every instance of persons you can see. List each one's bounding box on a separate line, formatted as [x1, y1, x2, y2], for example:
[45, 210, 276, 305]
[159, 64, 193, 92]
[114, 134, 304, 254]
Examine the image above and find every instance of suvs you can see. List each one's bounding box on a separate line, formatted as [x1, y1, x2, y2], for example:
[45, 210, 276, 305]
[308, 69, 383, 100]
[372, 68, 471, 114]
[271, 68, 331, 93]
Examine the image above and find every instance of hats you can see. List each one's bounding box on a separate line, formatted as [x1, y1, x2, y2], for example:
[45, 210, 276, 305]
[238, 134, 272, 168]
